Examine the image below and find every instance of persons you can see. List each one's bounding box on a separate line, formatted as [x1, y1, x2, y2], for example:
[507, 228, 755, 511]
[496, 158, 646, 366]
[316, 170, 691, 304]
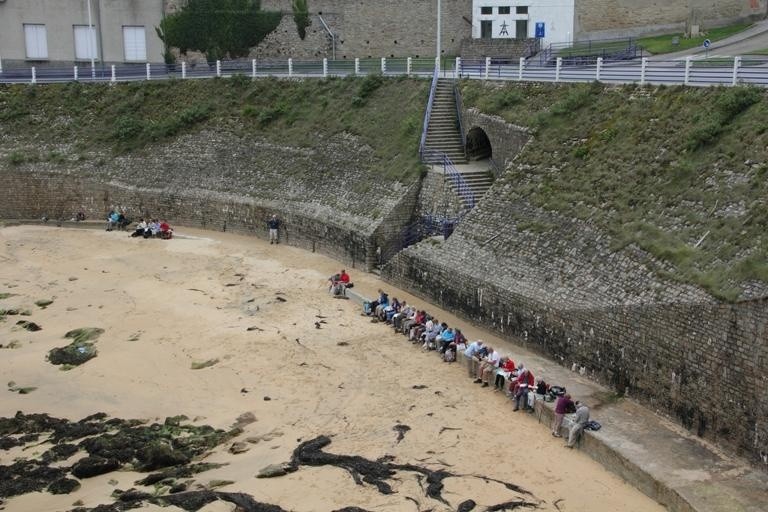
[333, 270, 350, 296]
[130, 218, 170, 239]
[268, 214, 281, 244]
[367, 287, 589, 448]
[105, 211, 126, 231]
